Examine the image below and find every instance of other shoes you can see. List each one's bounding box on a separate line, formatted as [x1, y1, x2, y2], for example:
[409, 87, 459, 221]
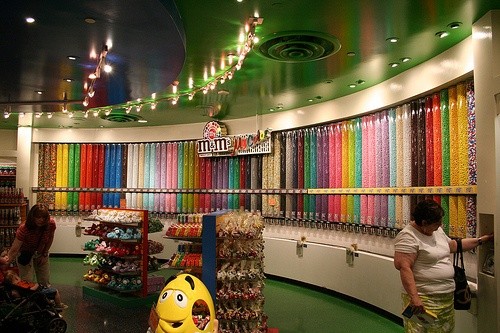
[48, 300, 69, 309]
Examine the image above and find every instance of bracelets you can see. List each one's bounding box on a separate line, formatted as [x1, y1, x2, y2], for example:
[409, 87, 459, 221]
[478, 237, 482, 245]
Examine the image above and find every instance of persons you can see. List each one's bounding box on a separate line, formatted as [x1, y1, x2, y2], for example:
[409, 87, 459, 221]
[0, 246, 68, 310]
[8, 203, 57, 284]
[394, 199, 493, 333]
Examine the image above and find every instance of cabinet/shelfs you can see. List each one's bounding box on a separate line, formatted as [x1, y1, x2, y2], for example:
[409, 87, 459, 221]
[79, 207, 164, 309]
[0, 166, 16, 189]
[157, 210, 267, 333]
[0, 196, 29, 276]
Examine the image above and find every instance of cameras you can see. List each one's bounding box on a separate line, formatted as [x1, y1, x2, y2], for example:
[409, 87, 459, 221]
[401, 302, 416, 319]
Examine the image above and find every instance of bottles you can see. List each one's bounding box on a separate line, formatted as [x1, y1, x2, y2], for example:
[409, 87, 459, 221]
[0, 187, 25, 248]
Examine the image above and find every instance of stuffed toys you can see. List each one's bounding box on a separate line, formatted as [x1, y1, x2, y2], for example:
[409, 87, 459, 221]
[81, 209, 142, 292]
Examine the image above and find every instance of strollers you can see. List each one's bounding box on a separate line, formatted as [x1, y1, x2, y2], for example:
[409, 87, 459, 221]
[0, 270, 67, 333]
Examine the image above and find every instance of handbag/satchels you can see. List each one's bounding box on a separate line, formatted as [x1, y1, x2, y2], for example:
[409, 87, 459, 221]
[451, 237, 471, 310]
[17, 250, 34, 266]
